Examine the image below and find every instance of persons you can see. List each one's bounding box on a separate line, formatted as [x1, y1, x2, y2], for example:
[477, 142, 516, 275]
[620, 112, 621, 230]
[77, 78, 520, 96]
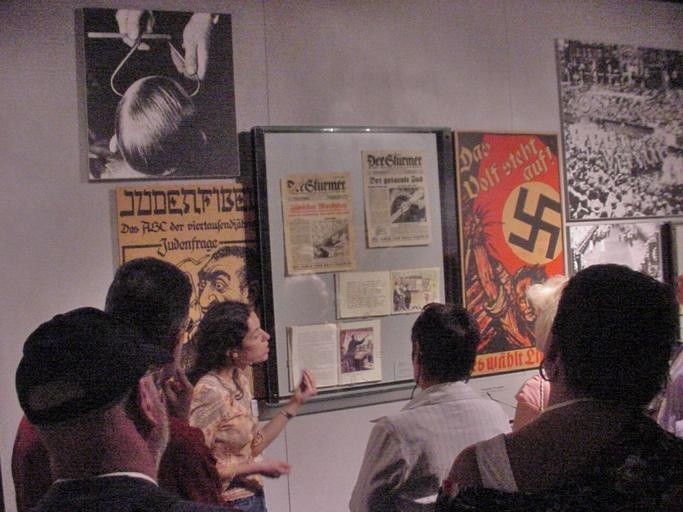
[188, 299, 319, 511]
[435, 261, 681, 510]
[177, 245, 262, 385]
[558, 40, 683, 218]
[13, 306, 244, 511]
[114, 10, 214, 85]
[514, 273, 577, 435]
[394, 278, 411, 311]
[346, 335, 368, 370]
[345, 303, 513, 512]
[9, 256, 226, 512]
[464, 218, 548, 349]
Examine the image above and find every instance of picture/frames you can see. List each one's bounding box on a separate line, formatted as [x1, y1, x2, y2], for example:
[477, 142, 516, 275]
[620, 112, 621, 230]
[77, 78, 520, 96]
[251, 125, 571, 405]
[668, 221, 683, 346]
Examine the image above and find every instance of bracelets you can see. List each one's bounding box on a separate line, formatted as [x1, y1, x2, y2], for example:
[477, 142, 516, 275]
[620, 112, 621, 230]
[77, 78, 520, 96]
[278, 408, 293, 419]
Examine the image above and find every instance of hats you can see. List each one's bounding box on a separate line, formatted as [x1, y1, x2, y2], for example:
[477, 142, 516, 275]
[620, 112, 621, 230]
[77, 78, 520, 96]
[16, 306, 175, 424]
